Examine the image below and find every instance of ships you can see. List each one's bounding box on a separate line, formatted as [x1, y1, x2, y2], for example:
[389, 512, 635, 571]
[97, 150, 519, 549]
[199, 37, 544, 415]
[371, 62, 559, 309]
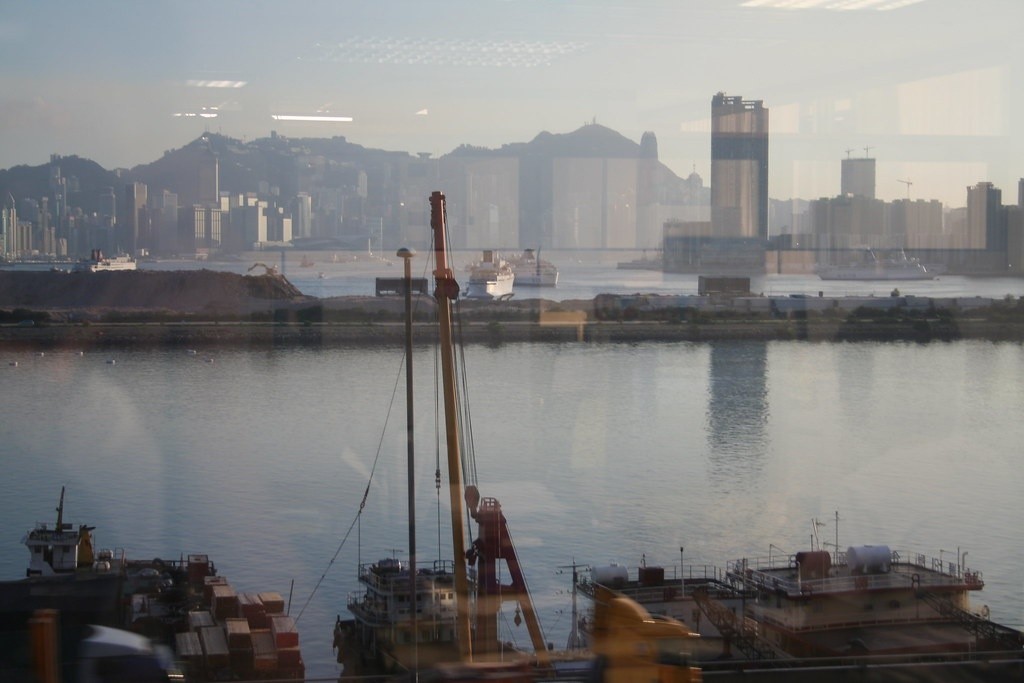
[820, 241, 937, 279]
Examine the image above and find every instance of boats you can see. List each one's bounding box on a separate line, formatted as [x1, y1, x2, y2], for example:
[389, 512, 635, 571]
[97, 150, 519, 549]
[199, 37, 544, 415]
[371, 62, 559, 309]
[467, 249, 515, 298]
[71, 249, 138, 272]
[506, 248, 559, 287]
[334, 192, 569, 680]
[19, 484, 218, 578]
